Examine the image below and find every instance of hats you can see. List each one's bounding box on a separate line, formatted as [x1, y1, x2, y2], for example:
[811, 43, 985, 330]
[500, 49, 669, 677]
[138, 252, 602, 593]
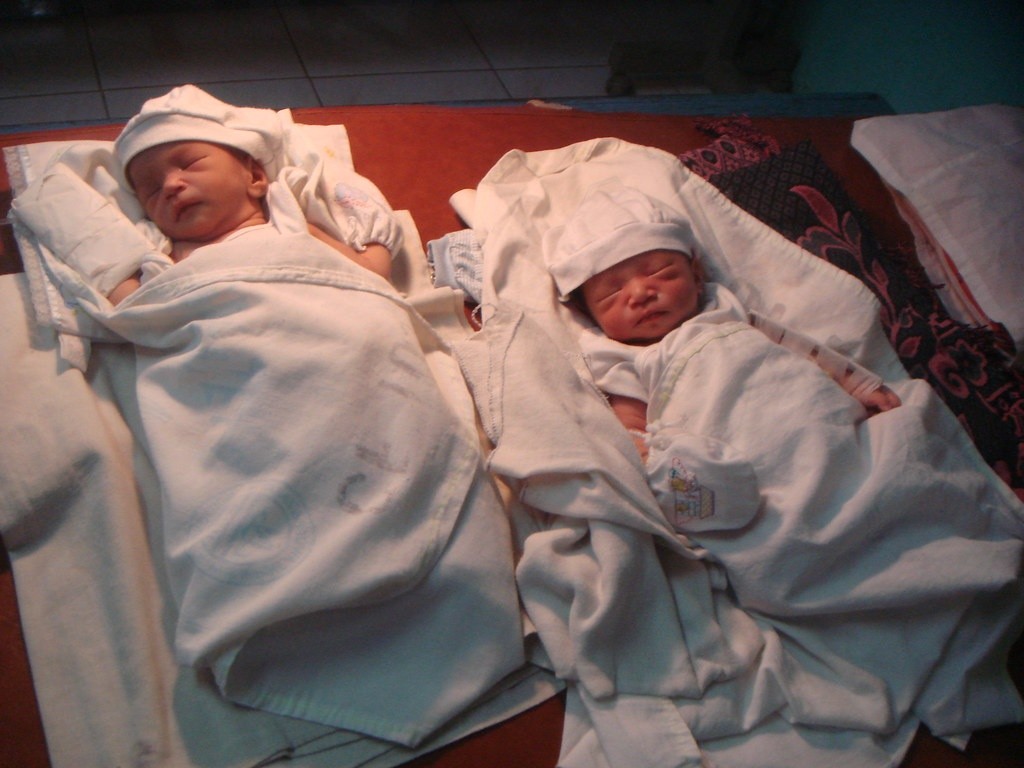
[542, 187, 698, 300]
[110, 84, 286, 194]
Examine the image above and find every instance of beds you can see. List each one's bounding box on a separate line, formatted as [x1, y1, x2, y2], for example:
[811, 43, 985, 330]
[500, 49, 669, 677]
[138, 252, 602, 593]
[0, 90, 1024, 768]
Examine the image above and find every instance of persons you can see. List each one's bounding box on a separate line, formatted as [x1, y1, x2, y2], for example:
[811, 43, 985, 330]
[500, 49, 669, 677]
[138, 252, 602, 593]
[90, 85, 393, 312]
[556, 187, 902, 478]
